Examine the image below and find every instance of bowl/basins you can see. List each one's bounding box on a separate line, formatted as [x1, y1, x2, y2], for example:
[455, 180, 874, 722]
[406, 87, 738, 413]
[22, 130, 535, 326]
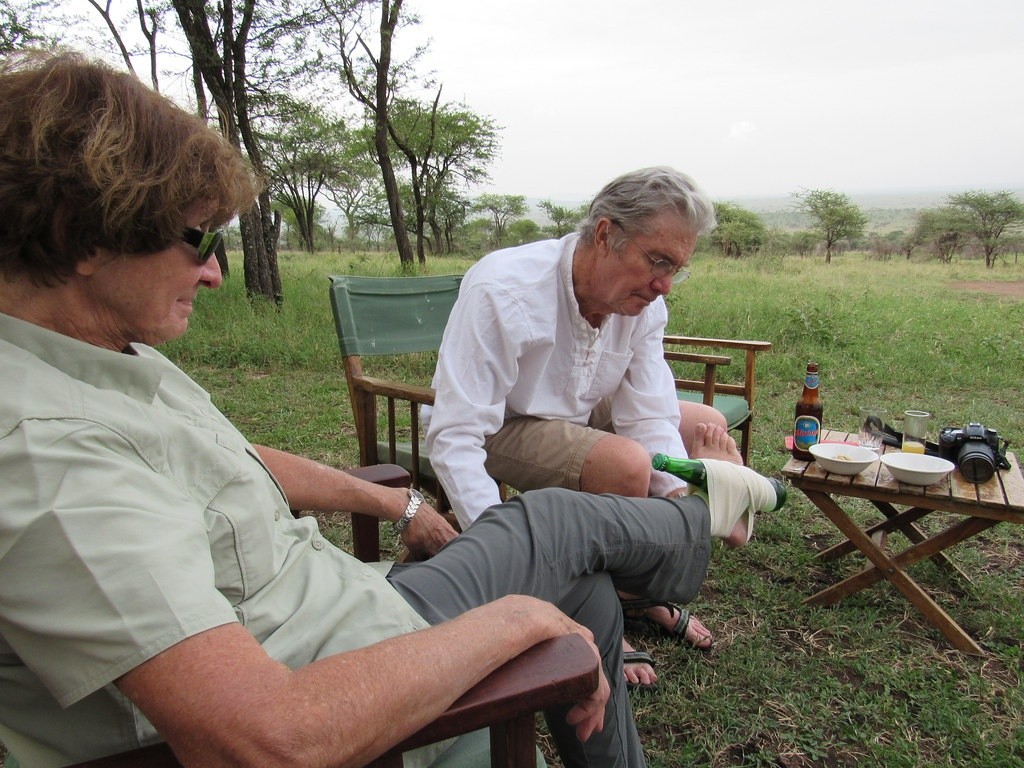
[880, 452, 955, 485]
[808, 443, 878, 474]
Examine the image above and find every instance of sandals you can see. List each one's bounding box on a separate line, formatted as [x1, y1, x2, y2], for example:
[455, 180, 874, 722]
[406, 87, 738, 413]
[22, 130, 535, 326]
[622, 651, 658, 691]
[624, 596, 713, 651]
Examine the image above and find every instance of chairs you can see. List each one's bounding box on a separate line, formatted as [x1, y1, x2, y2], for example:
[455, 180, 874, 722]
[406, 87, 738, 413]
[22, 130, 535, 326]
[62, 463, 599, 768]
[329, 274, 771, 566]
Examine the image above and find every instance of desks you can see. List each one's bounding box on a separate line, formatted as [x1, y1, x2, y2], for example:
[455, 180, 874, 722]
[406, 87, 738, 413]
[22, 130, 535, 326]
[779, 428, 1024, 656]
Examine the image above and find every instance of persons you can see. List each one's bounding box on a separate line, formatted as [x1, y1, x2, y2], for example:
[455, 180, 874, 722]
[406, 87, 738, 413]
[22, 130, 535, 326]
[420, 168, 730, 686]
[0, 60, 752, 768]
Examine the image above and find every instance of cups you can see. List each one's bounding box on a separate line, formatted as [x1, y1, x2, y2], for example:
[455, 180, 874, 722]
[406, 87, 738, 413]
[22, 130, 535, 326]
[860, 406, 887, 451]
[902, 410, 930, 455]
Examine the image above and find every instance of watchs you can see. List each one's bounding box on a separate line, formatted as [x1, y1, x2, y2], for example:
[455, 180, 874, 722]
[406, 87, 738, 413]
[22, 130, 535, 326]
[392, 488, 425, 533]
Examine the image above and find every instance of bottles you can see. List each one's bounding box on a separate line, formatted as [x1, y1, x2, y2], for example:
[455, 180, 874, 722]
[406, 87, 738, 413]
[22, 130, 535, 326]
[651, 452, 787, 512]
[793, 363, 823, 462]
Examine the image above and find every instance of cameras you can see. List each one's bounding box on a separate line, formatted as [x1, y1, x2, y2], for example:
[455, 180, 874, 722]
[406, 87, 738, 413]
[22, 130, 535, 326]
[940, 423, 999, 484]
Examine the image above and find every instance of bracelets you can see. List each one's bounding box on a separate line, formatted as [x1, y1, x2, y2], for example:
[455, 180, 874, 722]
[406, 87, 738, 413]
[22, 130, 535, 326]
[675, 492, 688, 498]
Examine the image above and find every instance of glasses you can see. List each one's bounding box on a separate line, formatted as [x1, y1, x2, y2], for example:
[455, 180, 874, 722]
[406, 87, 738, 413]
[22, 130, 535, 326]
[614, 220, 692, 284]
[176, 224, 225, 266]
